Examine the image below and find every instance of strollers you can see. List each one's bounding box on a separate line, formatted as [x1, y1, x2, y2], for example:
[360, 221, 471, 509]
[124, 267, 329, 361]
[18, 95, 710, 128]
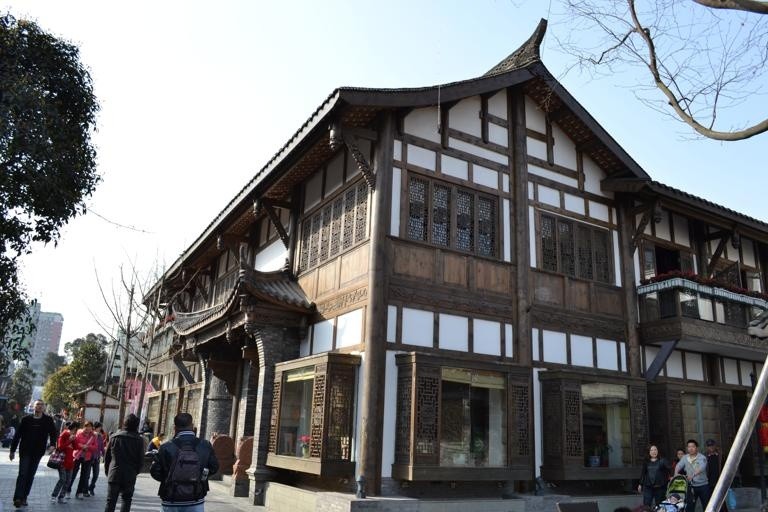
[1, 427, 15, 448]
[653, 474, 693, 512]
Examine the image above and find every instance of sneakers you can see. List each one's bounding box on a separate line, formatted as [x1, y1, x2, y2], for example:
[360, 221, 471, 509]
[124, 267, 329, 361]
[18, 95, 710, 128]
[48, 487, 95, 504]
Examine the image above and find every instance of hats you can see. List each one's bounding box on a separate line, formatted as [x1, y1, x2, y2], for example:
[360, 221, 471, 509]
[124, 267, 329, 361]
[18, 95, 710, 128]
[705, 438, 716, 445]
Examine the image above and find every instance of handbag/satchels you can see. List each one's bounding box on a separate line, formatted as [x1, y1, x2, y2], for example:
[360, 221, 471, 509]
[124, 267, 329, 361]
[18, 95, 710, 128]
[46, 447, 66, 470]
[724, 488, 737, 511]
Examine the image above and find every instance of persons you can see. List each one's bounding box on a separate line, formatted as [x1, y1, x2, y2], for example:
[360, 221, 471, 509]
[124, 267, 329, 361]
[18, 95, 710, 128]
[48, 414, 85, 444]
[103, 413, 145, 512]
[672, 448, 686, 474]
[701, 438, 728, 512]
[147, 432, 165, 451]
[656, 493, 681, 512]
[49, 421, 80, 504]
[64, 420, 99, 499]
[150, 412, 220, 512]
[138, 420, 154, 442]
[8, 399, 58, 508]
[636, 444, 673, 507]
[83, 421, 106, 498]
[672, 438, 712, 511]
[1, 423, 16, 448]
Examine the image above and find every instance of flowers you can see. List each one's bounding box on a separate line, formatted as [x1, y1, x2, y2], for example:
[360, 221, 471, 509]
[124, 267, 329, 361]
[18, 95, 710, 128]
[701, 276, 768, 301]
[639, 269, 698, 284]
[296, 435, 310, 450]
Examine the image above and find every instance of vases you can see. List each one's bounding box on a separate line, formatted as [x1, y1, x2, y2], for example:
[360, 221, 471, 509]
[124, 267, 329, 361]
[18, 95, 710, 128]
[588, 455, 601, 467]
[302, 447, 309, 458]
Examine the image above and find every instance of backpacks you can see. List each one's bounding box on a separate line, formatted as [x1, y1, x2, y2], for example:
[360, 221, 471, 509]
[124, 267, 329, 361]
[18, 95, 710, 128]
[157, 437, 209, 502]
